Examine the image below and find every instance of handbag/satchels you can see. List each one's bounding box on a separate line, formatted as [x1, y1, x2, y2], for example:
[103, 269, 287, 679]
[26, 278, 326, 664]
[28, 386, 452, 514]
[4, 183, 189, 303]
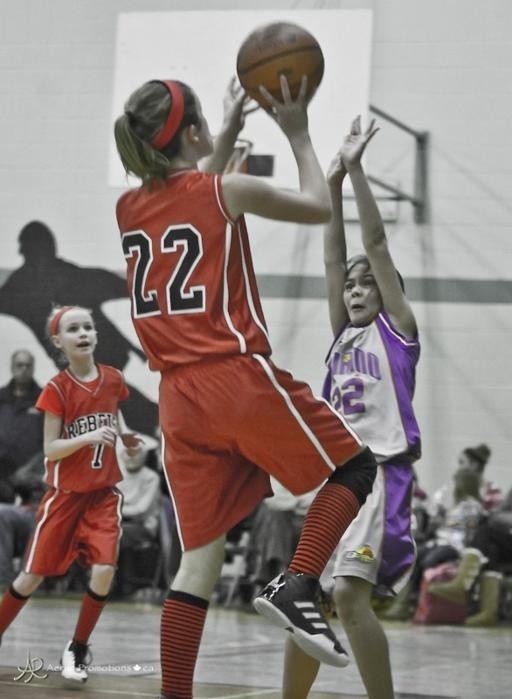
[415, 565, 468, 625]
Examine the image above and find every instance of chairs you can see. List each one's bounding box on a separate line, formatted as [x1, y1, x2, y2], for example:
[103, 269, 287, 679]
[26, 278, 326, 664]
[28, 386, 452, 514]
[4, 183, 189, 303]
[123, 539, 163, 595]
[215, 500, 269, 608]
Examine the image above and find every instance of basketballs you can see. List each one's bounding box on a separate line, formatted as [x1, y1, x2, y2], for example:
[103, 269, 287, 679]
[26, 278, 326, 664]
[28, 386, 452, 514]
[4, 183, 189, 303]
[236, 23, 324, 107]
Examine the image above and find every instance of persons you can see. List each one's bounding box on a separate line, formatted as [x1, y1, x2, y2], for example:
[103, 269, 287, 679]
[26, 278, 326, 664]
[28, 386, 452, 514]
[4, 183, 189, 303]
[282, 112, 420, 699]
[3, 347, 511, 628]
[1, 304, 149, 684]
[110, 73, 379, 699]
[2, 351, 182, 598]
[230, 501, 294, 614]
[382, 442, 511, 627]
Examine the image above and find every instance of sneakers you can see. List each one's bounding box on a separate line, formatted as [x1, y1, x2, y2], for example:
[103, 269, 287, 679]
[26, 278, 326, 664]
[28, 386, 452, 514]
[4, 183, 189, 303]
[255, 569, 349, 666]
[60, 640, 88, 682]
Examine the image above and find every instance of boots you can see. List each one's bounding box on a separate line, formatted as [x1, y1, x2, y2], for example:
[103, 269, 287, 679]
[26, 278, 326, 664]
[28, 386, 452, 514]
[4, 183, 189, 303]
[428, 547, 488, 605]
[381, 580, 416, 620]
[466, 570, 502, 625]
